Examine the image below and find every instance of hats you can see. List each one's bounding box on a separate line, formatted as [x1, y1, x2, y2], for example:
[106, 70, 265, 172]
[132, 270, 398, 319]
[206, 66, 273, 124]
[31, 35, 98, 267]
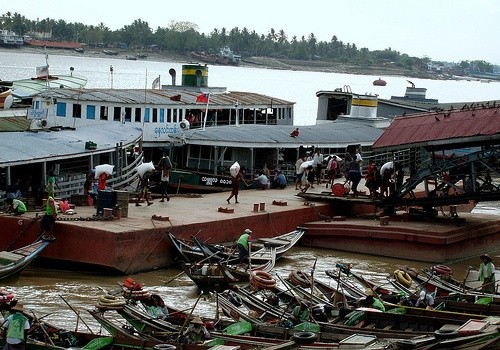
[420, 290, 426, 300]
[306, 150, 310, 154]
[245, 229, 252, 233]
[480, 254, 493, 262]
[11, 303, 28, 311]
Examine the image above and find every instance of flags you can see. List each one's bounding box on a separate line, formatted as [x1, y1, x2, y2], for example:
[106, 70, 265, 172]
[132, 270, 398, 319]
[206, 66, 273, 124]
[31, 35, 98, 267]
[152, 77, 159, 90]
[36, 66, 48, 76]
[196, 94, 209, 102]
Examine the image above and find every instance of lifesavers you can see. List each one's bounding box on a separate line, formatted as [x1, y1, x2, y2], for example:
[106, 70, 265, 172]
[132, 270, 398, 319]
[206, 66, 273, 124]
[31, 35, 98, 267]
[394, 269, 412, 288]
[430, 264, 453, 278]
[0, 289, 13, 302]
[289, 270, 313, 287]
[248, 270, 277, 289]
[97, 296, 126, 310]
[121, 279, 149, 300]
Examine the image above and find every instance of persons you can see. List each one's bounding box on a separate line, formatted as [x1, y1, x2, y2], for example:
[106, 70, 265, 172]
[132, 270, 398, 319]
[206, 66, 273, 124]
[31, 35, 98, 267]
[237, 229, 252, 265]
[295, 150, 338, 194]
[135, 168, 170, 206]
[365, 295, 385, 312]
[144, 297, 168, 318]
[30, 119, 50, 131]
[343, 153, 404, 199]
[477, 254, 495, 292]
[416, 291, 436, 308]
[252, 169, 287, 189]
[293, 300, 308, 321]
[226, 172, 242, 204]
[185, 316, 209, 343]
[410, 163, 418, 178]
[83, 172, 109, 195]
[3, 303, 30, 350]
[42, 171, 57, 241]
[186, 111, 196, 121]
[290, 128, 299, 136]
[465, 174, 472, 192]
[5, 180, 27, 217]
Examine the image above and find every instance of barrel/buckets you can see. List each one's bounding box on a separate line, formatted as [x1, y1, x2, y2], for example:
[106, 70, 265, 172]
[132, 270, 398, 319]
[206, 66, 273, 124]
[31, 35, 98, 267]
[113, 190, 129, 218]
[96, 190, 114, 216]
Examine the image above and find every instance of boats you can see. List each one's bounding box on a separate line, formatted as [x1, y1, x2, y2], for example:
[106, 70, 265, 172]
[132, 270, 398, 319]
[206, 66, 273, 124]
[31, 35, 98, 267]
[166, 226, 306, 263]
[0, 72, 87, 108]
[0, 118, 147, 206]
[0, 233, 56, 279]
[33, 87, 298, 149]
[183, 241, 280, 290]
[168, 116, 393, 192]
[2, 266, 500, 350]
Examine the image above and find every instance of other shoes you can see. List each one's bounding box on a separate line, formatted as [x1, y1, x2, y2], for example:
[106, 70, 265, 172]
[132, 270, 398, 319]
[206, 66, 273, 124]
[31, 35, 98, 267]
[295, 186, 315, 193]
